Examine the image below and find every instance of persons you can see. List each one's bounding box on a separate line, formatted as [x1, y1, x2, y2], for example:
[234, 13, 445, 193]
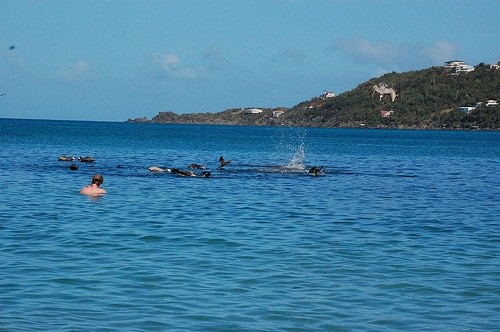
[80, 174, 107, 196]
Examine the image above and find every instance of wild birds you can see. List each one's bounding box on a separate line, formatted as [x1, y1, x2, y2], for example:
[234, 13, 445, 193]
[77, 156, 95, 163]
[197, 167, 211, 178]
[217, 156, 234, 169]
[370, 82, 397, 103]
[188, 164, 206, 170]
[58, 155, 75, 162]
[148, 166, 171, 174]
[171, 168, 197, 177]
[70, 164, 78, 170]
[308, 165, 327, 177]
